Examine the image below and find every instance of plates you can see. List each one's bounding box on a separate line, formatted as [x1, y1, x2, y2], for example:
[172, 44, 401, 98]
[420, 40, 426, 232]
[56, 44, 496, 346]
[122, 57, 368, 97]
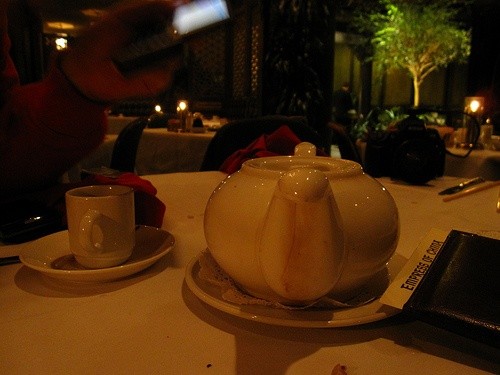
[184, 247, 409, 328]
[19, 225, 175, 284]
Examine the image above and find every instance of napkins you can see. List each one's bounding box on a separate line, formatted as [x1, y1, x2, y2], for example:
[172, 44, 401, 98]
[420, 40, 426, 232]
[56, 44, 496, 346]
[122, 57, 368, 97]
[63, 165, 165, 228]
[220, 124, 329, 175]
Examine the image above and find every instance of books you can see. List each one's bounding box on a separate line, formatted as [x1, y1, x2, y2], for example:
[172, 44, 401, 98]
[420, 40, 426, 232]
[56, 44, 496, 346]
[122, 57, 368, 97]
[379, 229, 500, 340]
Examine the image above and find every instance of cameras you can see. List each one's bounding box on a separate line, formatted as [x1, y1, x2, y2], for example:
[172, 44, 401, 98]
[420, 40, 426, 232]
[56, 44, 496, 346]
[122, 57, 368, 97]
[364, 105, 445, 187]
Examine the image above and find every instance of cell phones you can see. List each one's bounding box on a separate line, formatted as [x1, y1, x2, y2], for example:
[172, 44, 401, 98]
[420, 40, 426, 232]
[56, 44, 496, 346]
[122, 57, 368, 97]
[120, 0, 236, 70]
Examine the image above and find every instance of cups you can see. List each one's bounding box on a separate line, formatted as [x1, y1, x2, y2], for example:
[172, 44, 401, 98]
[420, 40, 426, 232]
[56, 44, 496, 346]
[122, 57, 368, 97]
[66, 185, 134, 268]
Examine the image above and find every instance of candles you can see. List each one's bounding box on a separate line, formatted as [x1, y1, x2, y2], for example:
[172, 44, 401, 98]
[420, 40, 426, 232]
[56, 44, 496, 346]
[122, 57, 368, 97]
[480, 117, 493, 152]
[178, 100, 188, 128]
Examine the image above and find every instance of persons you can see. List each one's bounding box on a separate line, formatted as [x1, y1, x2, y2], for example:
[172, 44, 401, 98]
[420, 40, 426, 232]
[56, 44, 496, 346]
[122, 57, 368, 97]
[332, 81, 355, 110]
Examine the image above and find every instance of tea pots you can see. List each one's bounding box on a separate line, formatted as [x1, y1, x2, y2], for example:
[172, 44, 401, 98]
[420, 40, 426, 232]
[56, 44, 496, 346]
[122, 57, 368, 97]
[203, 142, 401, 306]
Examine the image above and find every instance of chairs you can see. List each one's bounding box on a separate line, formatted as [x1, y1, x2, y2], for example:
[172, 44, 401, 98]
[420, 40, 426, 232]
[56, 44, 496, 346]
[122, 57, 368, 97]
[111, 116, 149, 177]
[201, 116, 366, 171]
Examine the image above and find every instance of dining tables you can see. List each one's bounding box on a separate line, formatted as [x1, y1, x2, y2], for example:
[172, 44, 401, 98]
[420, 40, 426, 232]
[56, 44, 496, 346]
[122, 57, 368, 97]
[0, 115, 500, 375]
